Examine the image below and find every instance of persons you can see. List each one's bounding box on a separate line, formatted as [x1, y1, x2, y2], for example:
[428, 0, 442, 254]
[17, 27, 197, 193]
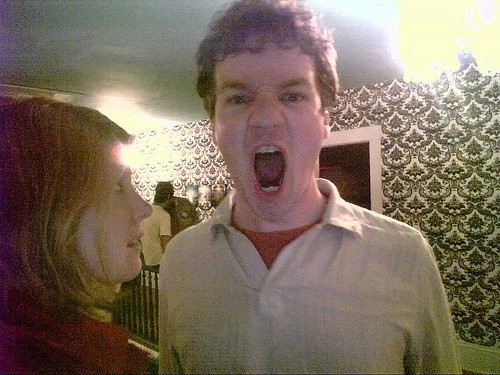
[155, 0, 466, 375]
[0, 90, 157, 375]
[137, 181, 173, 269]
